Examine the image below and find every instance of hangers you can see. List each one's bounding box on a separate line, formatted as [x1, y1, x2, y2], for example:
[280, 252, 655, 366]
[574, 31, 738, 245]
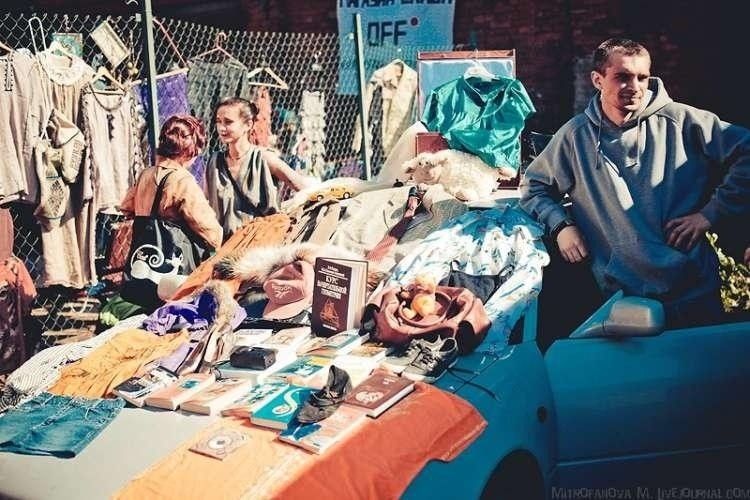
[464, 48, 495, 83]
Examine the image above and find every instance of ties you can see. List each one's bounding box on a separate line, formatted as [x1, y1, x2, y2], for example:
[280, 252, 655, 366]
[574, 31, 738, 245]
[363, 197, 418, 264]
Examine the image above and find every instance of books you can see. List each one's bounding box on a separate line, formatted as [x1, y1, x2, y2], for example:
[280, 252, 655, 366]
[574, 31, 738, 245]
[311, 256, 368, 338]
[145, 372, 216, 412]
[213, 326, 396, 379]
[179, 378, 251, 415]
[112, 366, 176, 408]
[189, 427, 253, 461]
[343, 372, 416, 418]
[222, 379, 290, 416]
[249, 384, 321, 427]
[273, 404, 366, 457]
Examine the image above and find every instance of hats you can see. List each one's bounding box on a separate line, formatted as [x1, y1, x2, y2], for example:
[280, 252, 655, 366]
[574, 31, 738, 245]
[263, 260, 314, 319]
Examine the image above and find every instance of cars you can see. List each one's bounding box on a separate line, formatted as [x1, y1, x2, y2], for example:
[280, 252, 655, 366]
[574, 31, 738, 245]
[0, 182, 750, 500]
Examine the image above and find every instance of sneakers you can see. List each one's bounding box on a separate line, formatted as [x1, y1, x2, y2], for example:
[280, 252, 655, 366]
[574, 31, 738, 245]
[379, 331, 458, 383]
[296, 365, 352, 423]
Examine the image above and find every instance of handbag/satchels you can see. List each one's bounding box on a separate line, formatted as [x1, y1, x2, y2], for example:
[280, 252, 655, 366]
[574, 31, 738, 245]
[120, 216, 210, 308]
[373, 286, 490, 353]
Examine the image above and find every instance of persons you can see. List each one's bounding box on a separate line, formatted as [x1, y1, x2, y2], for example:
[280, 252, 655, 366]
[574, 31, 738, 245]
[404, 149, 518, 205]
[120, 113, 224, 312]
[515, 35, 750, 331]
[204, 96, 307, 248]
